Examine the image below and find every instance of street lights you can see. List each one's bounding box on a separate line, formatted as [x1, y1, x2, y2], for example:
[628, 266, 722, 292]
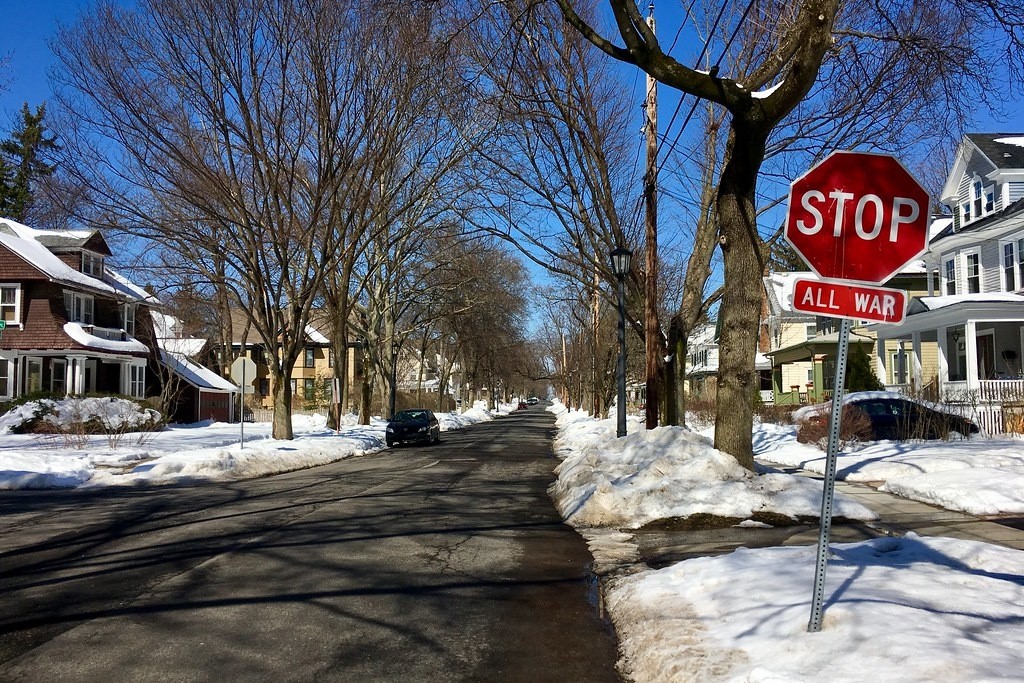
[610, 243, 634, 439]
[568, 370, 573, 413]
[388, 343, 400, 421]
[496, 379, 500, 412]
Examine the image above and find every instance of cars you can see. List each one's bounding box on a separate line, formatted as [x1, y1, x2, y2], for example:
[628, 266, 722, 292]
[528, 397, 538, 405]
[792, 391, 976, 441]
[518, 401, 527, 410]
[385, 407, 440, 447]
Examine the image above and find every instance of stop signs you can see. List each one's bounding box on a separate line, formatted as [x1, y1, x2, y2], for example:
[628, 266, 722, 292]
[781, 149, 932, 286]
